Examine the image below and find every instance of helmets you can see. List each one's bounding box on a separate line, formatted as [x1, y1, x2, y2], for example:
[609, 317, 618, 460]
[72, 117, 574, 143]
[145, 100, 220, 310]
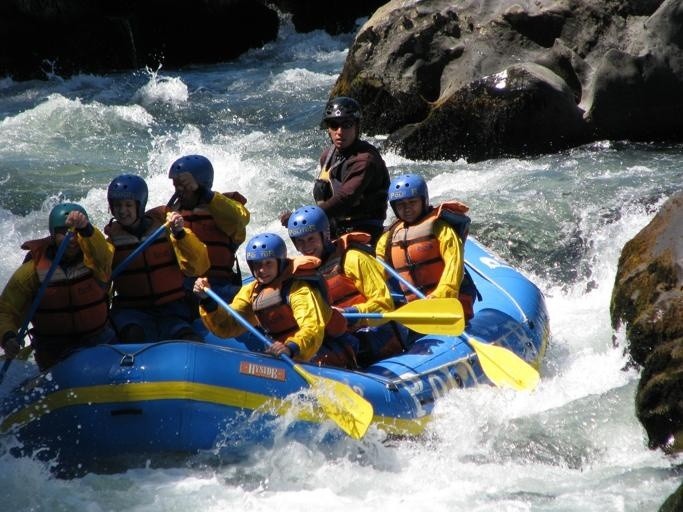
[388, 174, 429, 219]
[49, 203, 88, 243]
[246, 233, 287, 276]
[323, 97, 363, 134]
[169, 155, 213, 190]
[108, 174, 148, 218]
[288, 205, 330, 250]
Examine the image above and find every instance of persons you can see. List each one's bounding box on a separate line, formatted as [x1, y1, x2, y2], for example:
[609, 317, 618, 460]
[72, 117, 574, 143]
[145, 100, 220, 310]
[0, 202, 116, 371]
[192, 233, 368, 369]
[279, 98, 391, 250]
[286, 205, 402, 369]
[167, 154, 250, 320]
[374, 172, 474, 347]
[104, 175, 212, 345]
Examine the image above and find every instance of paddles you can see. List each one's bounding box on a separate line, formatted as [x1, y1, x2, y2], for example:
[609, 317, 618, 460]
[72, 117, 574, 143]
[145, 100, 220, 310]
[342, 298, 466, 337]
[376, 255, 541, 392]
[2, 212, 175, 361]
[199, 285, 374, 440]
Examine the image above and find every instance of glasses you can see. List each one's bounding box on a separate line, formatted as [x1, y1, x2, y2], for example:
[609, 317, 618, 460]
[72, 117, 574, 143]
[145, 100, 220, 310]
[326, 119, 356, 131]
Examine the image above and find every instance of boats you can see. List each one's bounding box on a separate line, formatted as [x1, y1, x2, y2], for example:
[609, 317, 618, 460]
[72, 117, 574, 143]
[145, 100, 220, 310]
[0, 226, 557, 477]
